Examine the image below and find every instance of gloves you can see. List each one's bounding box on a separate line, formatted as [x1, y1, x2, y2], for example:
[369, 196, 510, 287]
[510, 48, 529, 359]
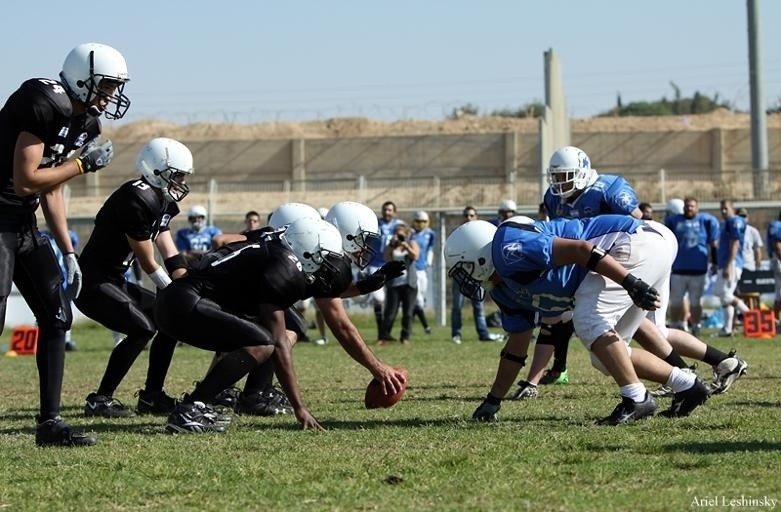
[62, 252, 82, 299]
[515, 379, 538, 403]
[75, 134, 114, 173]
[470, 398, 502, 422]
[357, 260, 406, 295]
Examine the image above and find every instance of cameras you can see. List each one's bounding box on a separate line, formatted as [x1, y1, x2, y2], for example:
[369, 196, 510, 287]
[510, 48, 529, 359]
[398, 234, 405, 242]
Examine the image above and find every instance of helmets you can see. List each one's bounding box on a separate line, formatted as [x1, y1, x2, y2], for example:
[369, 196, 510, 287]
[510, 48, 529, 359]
[59, 43, 130, 119]
[269, 201, 380, 284]
[666, 199, 684, 215]
[444, 220, 498, 301]
[547, 146, 591, 198]
[414, 211, 428, 221]
[136, 137, 193, 200]
[498, 200, 516, 212]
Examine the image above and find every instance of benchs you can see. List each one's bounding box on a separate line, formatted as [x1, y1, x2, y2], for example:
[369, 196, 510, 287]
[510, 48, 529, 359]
[734, 269, 780, 324]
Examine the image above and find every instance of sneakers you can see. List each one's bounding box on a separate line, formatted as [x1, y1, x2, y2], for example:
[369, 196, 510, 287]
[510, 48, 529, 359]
[541, 370, 566, 384]
[595, 347, 747, 425]
[34, 414, 95, 445]
[133, 379, 294, 434]
[84, 393, 136, 417]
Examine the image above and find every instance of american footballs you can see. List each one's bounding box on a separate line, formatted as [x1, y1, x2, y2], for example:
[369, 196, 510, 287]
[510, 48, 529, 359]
[365, 367, 409, 409]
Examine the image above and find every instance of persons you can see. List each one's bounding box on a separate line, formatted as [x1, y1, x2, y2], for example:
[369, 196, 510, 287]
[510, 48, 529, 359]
[0, 43, 781, 447]
